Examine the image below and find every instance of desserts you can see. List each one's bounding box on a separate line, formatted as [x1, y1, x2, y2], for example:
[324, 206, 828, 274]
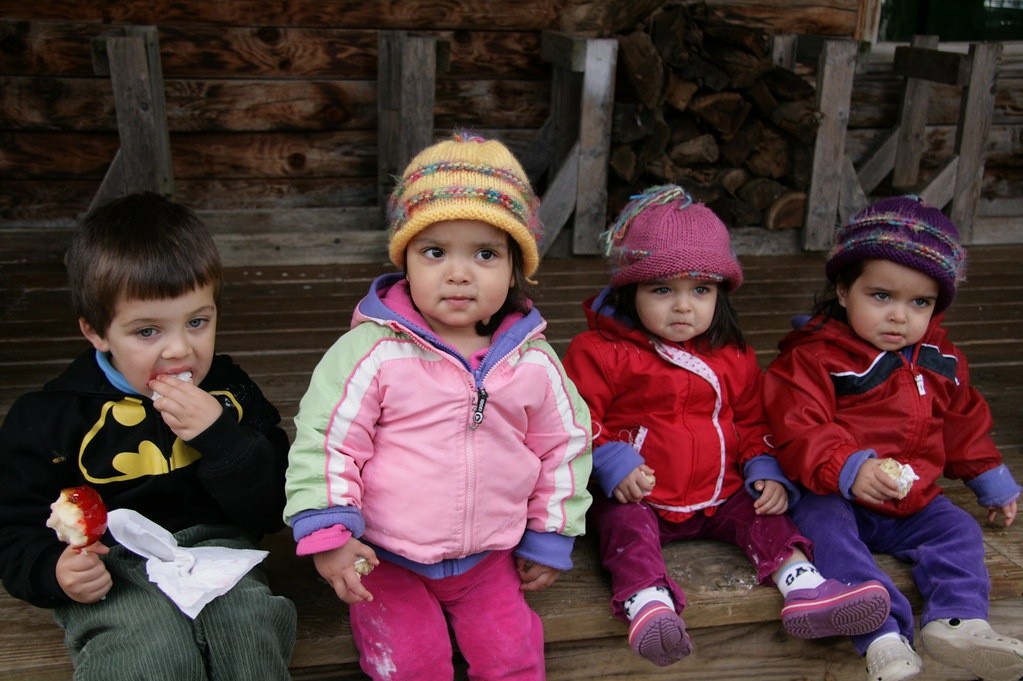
[878, 458, 918, 499]
[151, 371, 192, 401]
[46, 488, 108, 548]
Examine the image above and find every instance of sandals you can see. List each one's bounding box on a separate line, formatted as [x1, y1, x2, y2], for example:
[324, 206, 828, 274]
[781, 579, 891, 639]
[920, 617, 1023, 681]
[627, 600, 692, 669]
[865, 634, 923, 681]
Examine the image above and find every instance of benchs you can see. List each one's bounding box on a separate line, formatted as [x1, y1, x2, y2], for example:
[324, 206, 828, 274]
[0, 244, 1022, 681]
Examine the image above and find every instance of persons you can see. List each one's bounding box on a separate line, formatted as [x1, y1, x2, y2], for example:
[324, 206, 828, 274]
[282, 128, 593, 681]
[561, 182, 892, 667]
[0, 192, 290, 681]
[762, 195, 1023, 681]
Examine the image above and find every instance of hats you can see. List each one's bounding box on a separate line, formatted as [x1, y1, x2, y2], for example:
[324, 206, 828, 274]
[825, 194, 963, 318]
[599, 184, 743, 293]
[388, 130, 542, 286]
